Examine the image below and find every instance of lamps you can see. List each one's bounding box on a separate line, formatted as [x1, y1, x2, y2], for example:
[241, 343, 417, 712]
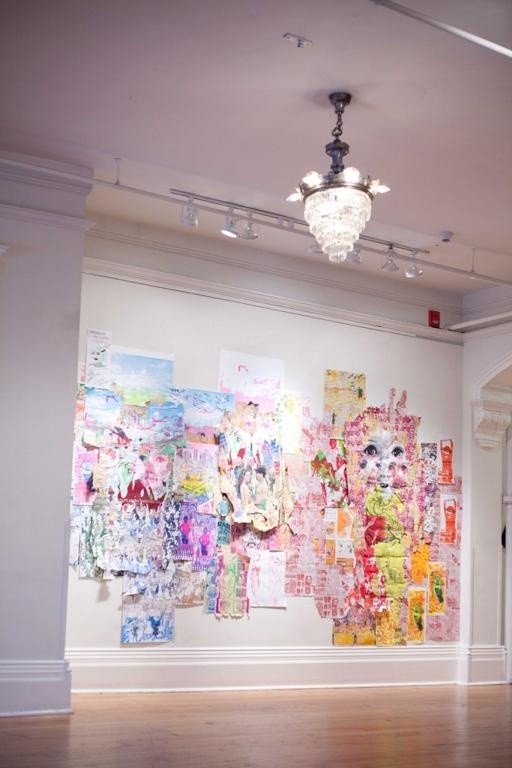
[282, 90, 391, 268]
[182, 195, 423, 281]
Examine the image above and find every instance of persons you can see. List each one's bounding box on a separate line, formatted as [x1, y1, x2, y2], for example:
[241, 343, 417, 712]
[255, 466, 270, 509]
[179, 515, 192, 545]
[237, 468, 253, 503]
[361, 427, 409, 499]
[139, 451, 169, 491]
[199, 526, 210, 556]
